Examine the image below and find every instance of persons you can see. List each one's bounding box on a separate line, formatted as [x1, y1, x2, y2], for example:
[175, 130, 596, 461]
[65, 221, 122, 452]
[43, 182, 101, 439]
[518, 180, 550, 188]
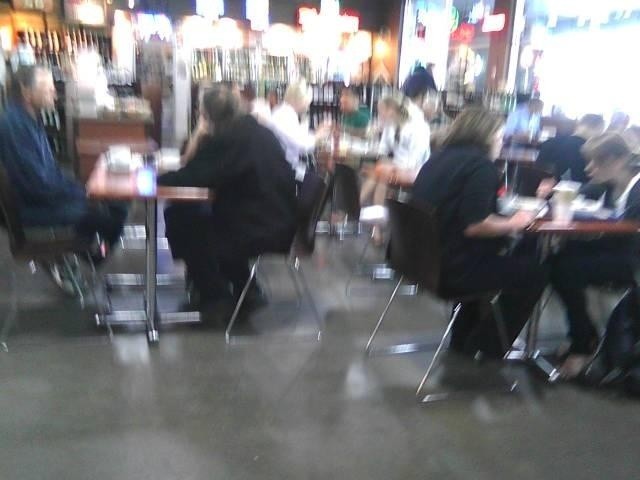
[0, 29, 305, 326]
[340, 92, 639, 385]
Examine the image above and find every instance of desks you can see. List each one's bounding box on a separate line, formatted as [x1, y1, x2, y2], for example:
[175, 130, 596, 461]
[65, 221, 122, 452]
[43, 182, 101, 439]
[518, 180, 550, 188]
[85, 145, 211, 346]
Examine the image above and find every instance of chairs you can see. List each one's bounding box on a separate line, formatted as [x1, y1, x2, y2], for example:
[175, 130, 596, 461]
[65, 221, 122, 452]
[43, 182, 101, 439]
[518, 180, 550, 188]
[2, 166, 113, 339]
[224, 166, 329, 345]
[333, 130, 639, 403]
